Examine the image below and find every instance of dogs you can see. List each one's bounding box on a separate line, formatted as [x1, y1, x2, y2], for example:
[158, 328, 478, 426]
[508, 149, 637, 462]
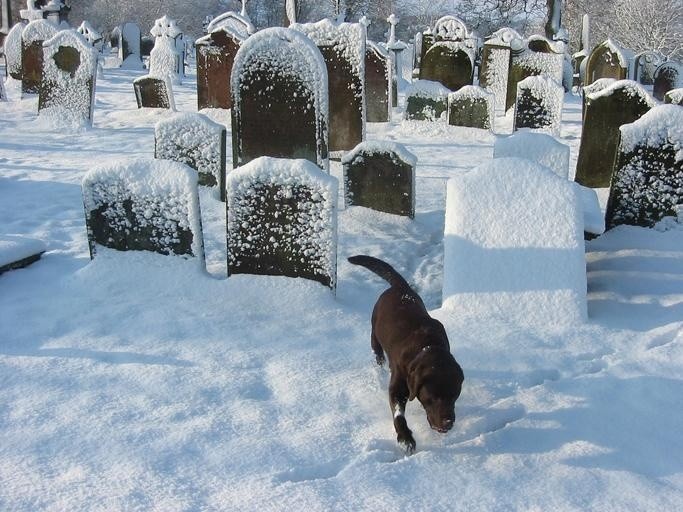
[347, 255, 464, 455]
